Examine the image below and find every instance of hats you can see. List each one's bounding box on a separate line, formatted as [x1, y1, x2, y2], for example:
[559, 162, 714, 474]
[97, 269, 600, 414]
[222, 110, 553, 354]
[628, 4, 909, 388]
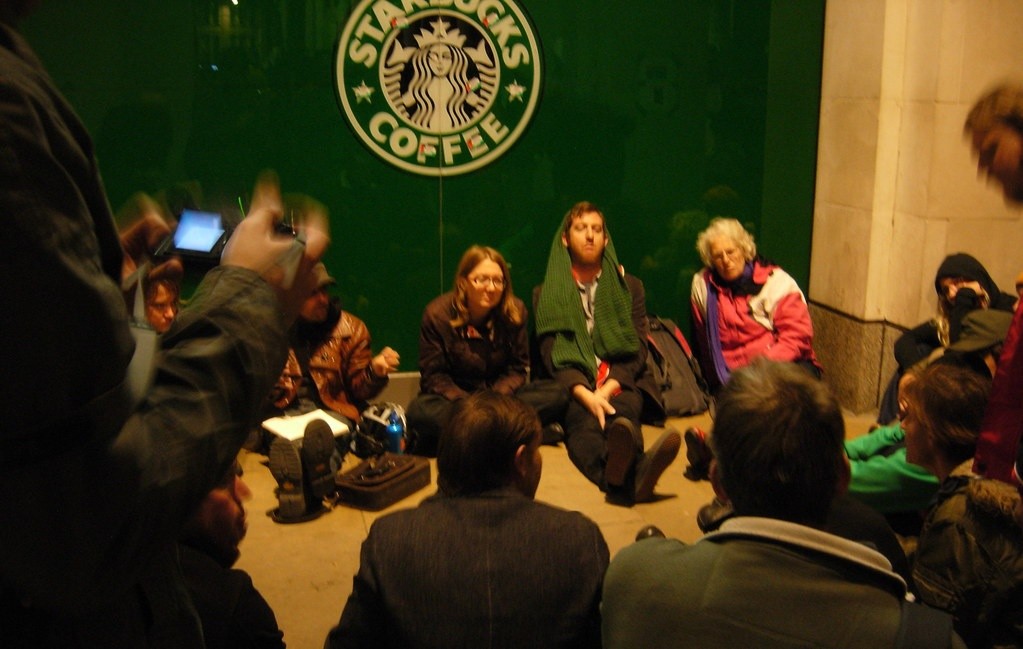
[306, 262, 340, 298]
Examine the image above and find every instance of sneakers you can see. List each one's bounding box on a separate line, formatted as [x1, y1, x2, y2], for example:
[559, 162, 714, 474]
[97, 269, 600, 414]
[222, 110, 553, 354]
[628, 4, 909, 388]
[630, 429, 680, 501]
[687, 426, 714, 476]
[604, 417, 636, 489]
[302, 418, 337, 504]
[268, 435, 310, 522]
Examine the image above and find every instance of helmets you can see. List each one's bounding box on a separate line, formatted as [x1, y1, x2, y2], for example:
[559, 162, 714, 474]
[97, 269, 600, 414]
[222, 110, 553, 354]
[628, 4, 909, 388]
[347, 404, 412, 461]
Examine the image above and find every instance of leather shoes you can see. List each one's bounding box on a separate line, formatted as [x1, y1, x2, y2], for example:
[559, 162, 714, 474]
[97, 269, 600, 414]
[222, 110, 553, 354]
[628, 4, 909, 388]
[538, 419, 567, 445]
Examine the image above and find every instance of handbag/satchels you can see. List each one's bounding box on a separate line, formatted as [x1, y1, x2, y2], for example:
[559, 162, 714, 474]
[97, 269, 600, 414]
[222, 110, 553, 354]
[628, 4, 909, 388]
[333, 444, 432, 511]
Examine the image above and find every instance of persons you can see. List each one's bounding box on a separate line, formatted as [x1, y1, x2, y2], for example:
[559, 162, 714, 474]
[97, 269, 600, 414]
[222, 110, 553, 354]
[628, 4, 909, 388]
[601, 354, 971, 648]
[684, 215, 822, 471]
[908, 362, 1023, 648]
[697, 347, 995, 535]
[0, 0, 328, 648]
[323, 389, 609, 649]
[868, 252, 1018, 435]
[634, 488, 921, 600]
[405, 245, 571, 443]
[125, 275, 252, 499]
[242, 263, 400, 521]
[963, 80, 1023, 489]
[532, 199, 681, 503]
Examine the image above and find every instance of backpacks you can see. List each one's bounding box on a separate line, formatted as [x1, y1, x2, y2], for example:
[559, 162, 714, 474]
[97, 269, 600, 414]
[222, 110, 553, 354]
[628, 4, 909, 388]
[640, 311, 712, 414]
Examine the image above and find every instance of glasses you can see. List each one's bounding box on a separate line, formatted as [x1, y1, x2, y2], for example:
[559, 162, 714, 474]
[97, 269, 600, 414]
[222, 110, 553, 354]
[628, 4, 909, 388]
[465, 271, 510, 291]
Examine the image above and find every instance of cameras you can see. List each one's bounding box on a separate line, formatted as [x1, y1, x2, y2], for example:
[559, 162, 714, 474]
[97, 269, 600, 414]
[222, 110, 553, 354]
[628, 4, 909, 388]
[148, 208, 294, 270]
